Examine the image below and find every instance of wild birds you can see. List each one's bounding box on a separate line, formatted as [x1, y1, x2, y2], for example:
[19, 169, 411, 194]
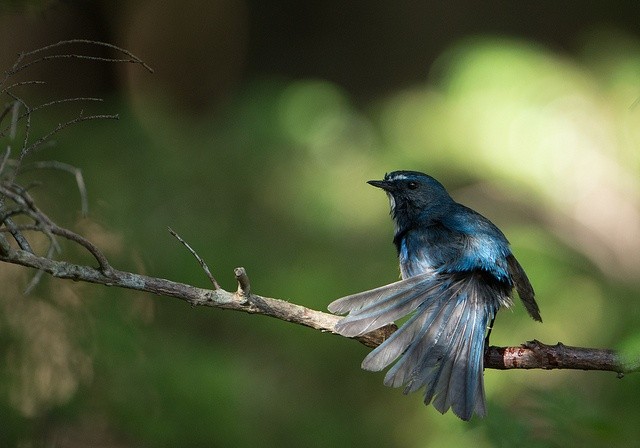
[327, 171, 540, 422]
